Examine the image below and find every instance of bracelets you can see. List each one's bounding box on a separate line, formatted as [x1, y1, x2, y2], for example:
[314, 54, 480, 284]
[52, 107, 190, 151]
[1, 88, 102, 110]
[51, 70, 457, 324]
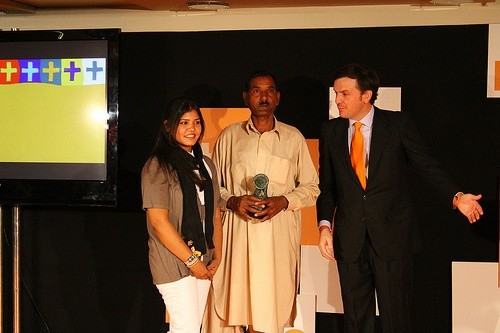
[184, 253, 199, 268]
[319, 226, 332, 234]
[452, 193, 462, 209]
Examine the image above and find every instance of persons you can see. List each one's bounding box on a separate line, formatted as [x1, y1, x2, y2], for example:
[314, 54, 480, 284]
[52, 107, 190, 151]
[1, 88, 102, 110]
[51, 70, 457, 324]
[201, 71, 322, 333]
[141, 100, 223, 333]
[316, 62, 484, 333]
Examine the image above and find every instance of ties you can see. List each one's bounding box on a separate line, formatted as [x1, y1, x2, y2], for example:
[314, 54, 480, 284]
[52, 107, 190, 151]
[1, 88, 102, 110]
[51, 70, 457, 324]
[350, 121, 366, 190]
